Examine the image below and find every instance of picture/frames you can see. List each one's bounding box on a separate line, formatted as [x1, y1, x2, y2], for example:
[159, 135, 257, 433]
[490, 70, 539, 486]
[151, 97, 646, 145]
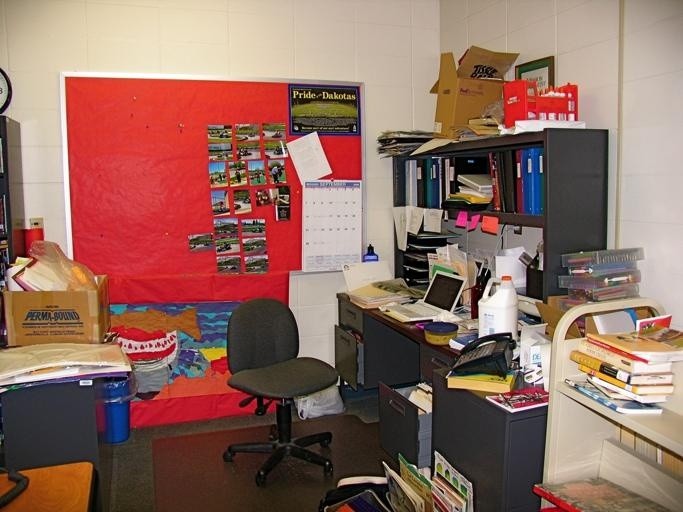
[423, 270, 467, 313]
[515, 55, 554, 90]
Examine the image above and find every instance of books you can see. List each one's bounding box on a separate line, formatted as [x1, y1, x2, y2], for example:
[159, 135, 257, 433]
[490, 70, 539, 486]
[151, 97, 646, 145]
[449, 334, 478, 350]
[382, 451, 473, 512]
[447, 371, 518, 394]
[486, 387, 550, 413]
[457, 173, 492, 193]
[5, 256, 97, 293]
[565, 331, 683, 415]
[459, 117, 499, 142]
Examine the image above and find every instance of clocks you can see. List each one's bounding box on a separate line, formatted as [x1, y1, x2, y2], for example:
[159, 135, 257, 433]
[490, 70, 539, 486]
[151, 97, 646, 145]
[0, 69, 12, 113]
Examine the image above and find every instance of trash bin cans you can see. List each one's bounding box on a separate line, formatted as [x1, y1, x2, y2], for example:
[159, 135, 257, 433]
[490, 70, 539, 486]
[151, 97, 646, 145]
[94, 370, 139, 445]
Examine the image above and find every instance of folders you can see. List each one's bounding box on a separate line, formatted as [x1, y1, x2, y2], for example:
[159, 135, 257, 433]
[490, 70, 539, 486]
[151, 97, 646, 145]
[489, 148, 543, 216]
[401, 157, 455, 209]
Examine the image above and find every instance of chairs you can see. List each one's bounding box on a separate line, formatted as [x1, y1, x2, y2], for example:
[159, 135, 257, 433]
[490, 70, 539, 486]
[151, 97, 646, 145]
[222, 297, 334, 488]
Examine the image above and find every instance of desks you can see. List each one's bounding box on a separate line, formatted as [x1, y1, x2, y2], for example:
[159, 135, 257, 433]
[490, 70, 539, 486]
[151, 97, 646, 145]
[333, 280, 548, 511]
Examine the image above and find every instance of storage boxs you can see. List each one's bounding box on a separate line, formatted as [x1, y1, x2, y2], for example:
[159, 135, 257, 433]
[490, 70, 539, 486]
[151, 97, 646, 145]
[432, 50, 506, 139]
[2, 274, 110, 345]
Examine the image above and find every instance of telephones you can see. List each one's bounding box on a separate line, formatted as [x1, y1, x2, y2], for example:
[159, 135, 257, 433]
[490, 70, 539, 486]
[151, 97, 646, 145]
[451, 331, 517, 379]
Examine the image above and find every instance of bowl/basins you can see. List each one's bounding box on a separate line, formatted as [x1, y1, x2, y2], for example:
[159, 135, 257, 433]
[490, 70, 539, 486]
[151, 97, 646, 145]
[423, 322, 459, 346]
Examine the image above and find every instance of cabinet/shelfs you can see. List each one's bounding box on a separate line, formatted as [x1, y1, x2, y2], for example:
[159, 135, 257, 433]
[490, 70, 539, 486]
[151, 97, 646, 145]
[379, 343, 455, 472]
[542, 298, 682, 512]
[0, 373, 103, 473]
[0, 115, 24, 276]
[391, 128, 610, 309]
[335, 296, 366, 391]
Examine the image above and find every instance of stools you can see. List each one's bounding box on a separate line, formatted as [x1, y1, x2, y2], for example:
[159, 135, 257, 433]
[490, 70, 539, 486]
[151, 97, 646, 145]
[0, 460, 104, 512]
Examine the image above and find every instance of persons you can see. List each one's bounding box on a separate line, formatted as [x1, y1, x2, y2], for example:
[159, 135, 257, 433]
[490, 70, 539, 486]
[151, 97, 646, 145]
[223, 242, 230, 249]
[235, 169, 242, 185]
[272, 164, 280, 183]
[254, 169, 262, 183]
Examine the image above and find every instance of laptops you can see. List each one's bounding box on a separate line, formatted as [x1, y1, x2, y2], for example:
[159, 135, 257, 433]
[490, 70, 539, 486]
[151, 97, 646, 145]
[382, 270, 467, 322]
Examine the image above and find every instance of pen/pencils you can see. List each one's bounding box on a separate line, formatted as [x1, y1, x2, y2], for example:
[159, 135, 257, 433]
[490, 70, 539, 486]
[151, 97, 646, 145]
[400, 299, 417, 305]
